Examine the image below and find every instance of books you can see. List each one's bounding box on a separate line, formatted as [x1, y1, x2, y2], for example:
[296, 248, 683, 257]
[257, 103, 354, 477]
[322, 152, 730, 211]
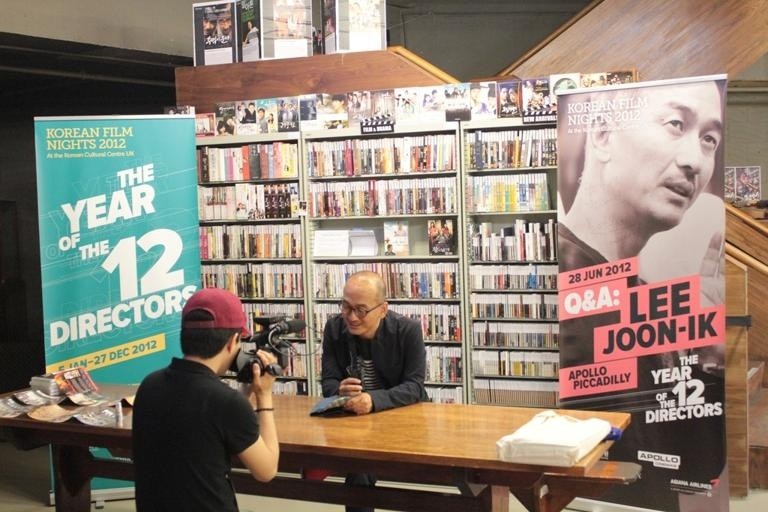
[0, 364, 134, 429]
[190, 0, 388, 67]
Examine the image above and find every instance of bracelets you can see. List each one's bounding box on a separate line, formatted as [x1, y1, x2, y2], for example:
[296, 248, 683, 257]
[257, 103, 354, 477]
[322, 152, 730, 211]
[252, 405, 276, 412]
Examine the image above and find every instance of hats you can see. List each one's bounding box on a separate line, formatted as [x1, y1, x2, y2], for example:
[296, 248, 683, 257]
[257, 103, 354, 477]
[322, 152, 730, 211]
[181, 287, 248, 337]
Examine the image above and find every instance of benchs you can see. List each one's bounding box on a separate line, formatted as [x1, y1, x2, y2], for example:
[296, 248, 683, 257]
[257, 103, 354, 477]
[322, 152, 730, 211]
[230, 451, 642, 512]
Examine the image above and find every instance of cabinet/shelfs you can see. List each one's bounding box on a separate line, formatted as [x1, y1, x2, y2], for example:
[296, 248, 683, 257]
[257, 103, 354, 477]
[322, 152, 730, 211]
[196, 117, 558, 409]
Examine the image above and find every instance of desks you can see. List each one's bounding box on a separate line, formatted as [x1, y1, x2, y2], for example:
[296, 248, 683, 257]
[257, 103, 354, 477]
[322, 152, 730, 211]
[0, 381, 632, 512]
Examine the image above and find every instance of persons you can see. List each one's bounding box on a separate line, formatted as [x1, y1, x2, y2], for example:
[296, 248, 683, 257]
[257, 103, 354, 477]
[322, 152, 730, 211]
[130, 285, 282, 511]
[320, 272, 432, 511]
[556, 78, 723, 482]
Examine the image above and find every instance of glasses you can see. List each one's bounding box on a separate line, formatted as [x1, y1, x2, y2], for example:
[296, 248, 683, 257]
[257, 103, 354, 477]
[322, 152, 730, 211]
[338, 299, 383, 318]
